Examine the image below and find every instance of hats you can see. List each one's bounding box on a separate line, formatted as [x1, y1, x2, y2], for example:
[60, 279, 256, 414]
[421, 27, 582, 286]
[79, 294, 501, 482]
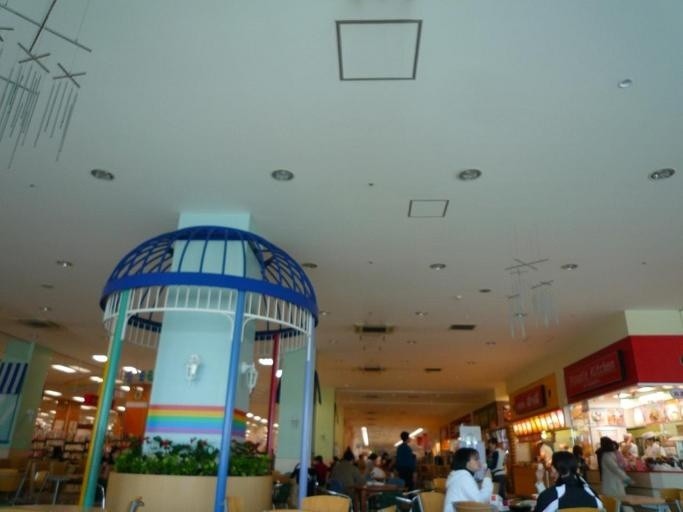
[594, 437, 619, 453]
[641, 431, 655, 440]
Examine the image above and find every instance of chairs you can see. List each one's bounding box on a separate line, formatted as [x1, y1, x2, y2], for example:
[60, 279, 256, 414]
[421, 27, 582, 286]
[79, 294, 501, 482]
[1, 447, 45, 494]
[303, 494, 350, 512]
[412, 475, 447, 511]
[662, 489, 682, 510]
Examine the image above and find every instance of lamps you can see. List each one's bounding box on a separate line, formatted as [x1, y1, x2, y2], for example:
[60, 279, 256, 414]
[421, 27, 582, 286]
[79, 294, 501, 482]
[185, 354, 201, 380]
[241, 362, 259, 395]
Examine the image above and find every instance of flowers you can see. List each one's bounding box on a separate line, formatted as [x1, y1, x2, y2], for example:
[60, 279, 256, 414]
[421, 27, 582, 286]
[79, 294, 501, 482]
[111, 435, 270, 476]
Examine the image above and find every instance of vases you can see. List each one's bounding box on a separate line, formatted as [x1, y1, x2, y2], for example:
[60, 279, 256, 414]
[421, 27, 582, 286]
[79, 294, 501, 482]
[101, 472, 273, 511]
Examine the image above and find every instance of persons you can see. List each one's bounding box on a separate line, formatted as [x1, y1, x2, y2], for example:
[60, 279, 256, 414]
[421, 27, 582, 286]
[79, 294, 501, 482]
[639, 431, 665, 462]
[308, 428, 454, 512]
[600, 440, 637, 512]
[529, 450, 604, 512]
[571, 444, 590, 481]
[484, 438, 506, 498]
[617, 433, 638, 458]
[594, 436, 613, 478]
[442, 446, 499, 511]
